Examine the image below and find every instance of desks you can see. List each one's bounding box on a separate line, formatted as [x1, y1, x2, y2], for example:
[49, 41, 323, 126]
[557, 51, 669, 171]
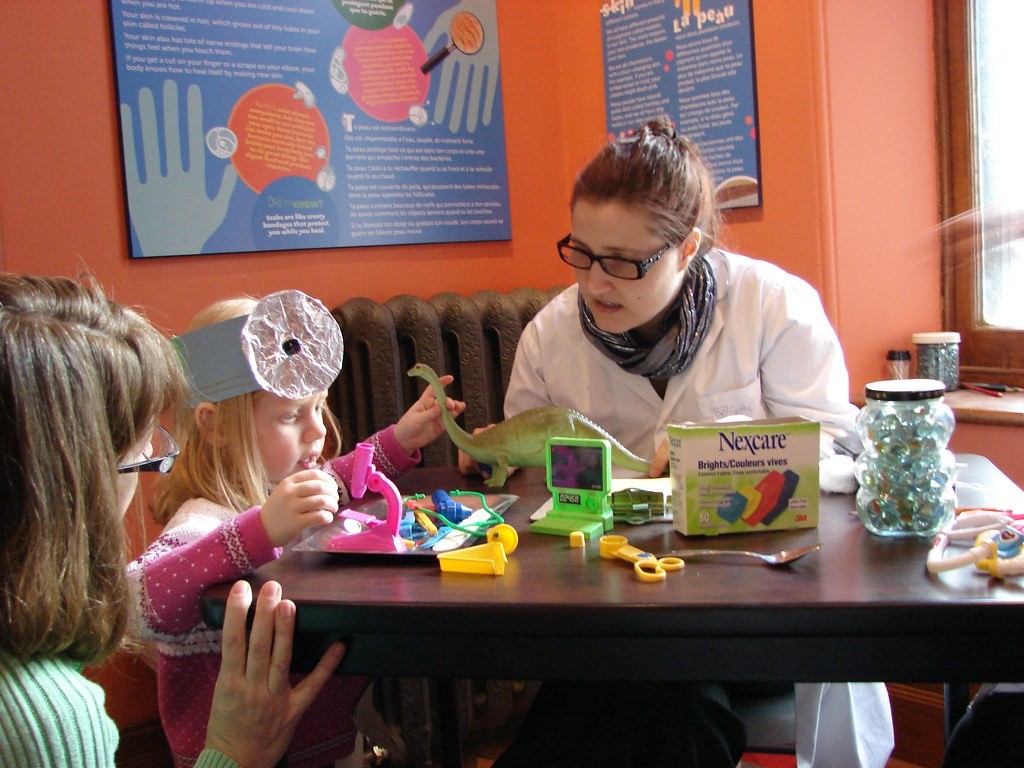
[197, 454, 1024, 768]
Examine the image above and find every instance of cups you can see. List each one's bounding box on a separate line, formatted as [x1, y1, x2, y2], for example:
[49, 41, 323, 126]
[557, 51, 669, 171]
[885, 350, 912, 379]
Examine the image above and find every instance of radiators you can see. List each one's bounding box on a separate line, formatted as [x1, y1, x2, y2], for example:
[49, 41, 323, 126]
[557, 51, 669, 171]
[327, 285, 568, 768]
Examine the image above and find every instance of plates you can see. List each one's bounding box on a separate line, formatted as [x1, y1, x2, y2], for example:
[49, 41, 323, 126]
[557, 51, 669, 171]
[292, 492, 521, 555]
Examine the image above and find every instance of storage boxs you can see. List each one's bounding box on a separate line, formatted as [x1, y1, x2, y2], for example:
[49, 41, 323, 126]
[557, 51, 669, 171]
[665, 415, 821, 536]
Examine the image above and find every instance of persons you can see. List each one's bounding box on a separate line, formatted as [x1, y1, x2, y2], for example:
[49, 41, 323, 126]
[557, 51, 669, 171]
[121, 294, 467, 767]
[451, 118, 868, 768]
[1, 270, 348, 768]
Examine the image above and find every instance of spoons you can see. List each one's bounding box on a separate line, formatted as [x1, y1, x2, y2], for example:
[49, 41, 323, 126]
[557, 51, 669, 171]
[655, 543, 822, 566]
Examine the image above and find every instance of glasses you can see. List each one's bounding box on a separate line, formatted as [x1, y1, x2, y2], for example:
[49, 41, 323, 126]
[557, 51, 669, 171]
[557, 232, 686, 280]
[115, 424, 179, 475]
